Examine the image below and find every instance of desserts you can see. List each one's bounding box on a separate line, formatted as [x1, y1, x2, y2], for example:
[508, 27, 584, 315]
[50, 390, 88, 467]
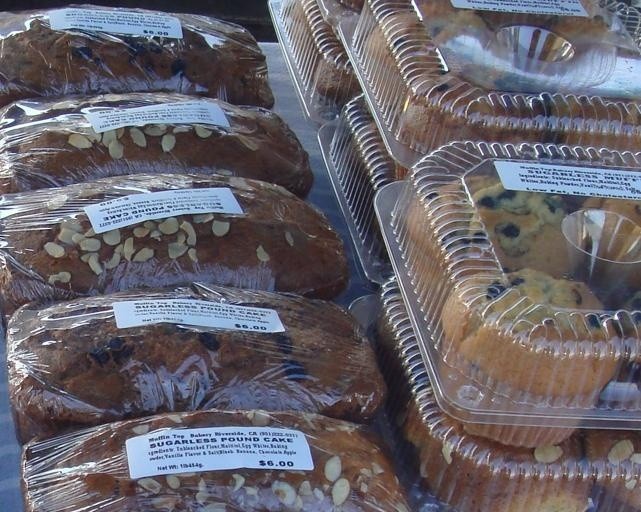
[273, 0, 641, 281]
[369, 153, 640, 512]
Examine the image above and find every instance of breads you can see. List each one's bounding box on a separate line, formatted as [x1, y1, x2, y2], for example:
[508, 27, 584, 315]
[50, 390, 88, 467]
[20, 409, 407, 511]
[1, 169, 350, 331]
[3, 283, 388, 444]
[0, 93, 314, 198]
[1, 4, 274, 109]
